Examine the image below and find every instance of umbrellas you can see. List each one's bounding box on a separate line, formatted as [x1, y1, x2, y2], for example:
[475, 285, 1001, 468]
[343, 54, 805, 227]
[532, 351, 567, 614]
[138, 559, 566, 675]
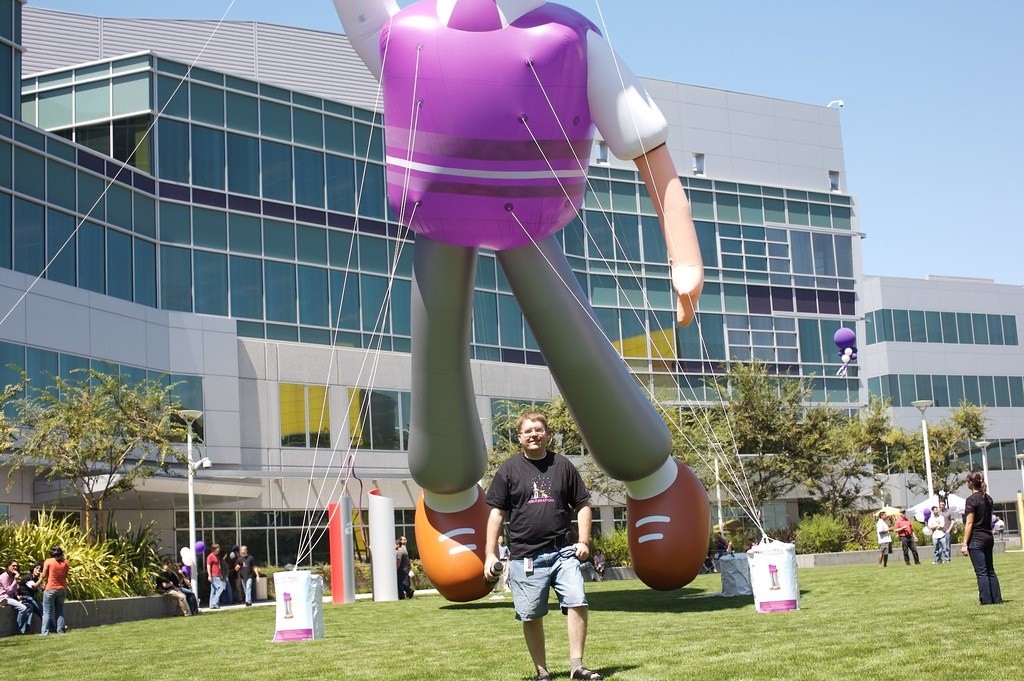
[873, 504, 902, 517]
[910, 494, 965, 514]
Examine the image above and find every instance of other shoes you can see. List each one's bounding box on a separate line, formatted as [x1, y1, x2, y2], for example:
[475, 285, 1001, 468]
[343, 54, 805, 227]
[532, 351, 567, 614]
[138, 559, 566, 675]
[410, 591, 414, 599]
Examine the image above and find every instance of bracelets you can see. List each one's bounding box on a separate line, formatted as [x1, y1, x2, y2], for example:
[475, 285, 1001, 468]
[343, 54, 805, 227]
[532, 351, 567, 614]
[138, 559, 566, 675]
[962, 545, 967, 547]
[579, 541, 589, 548]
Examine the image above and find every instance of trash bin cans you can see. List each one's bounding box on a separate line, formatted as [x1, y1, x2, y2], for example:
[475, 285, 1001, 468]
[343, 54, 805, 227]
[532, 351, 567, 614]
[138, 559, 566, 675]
[255, 577, 267, 600]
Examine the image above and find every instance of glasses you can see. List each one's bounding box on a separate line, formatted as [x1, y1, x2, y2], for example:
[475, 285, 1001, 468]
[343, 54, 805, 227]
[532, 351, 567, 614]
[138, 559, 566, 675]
[518, 429, 546, 437]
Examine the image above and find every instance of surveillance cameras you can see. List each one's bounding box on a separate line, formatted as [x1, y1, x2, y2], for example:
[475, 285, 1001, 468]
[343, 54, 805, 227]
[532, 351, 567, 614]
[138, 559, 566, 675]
[203, 460, 213, 468]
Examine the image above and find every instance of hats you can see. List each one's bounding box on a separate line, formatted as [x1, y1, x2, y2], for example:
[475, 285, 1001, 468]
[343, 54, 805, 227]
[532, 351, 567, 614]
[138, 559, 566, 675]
[900, 510, 906, 513]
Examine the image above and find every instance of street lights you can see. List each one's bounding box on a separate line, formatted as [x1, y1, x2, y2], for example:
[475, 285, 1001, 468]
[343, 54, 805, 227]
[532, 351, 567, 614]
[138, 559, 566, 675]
[177, 409, 213, 609]
[910, 399, 934, 512]
[975, 441, 991, 496]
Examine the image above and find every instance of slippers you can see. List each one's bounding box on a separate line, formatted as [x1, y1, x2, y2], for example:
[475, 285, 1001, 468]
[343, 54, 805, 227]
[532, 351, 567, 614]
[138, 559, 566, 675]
[573, 667, 603, 680]
[536, 675, 550, 681]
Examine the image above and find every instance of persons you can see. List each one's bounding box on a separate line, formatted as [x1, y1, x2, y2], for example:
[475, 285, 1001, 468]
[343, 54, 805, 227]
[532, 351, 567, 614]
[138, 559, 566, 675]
[594, 549, 605, 579]
[743, 539, 754, 551]
[0, 547, 68, 636]
[962, 470, 1002, 604]
[484, 411, 604, 681]
[156, 559, 201, 616]
[995, 516, 1005, 540]
[928, 500, 955, 564]
[705, 532, 733, 574]
[207, 543, 259, 608]
[493, 536, 511, 593]
[894, 510, 922, 565]
[877, 512, 893, 568]
[395, 537, 414, 600]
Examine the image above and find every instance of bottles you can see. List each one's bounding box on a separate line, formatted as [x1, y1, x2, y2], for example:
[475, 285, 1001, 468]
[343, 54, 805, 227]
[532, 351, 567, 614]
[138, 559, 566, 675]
[485, 561, 504, 587]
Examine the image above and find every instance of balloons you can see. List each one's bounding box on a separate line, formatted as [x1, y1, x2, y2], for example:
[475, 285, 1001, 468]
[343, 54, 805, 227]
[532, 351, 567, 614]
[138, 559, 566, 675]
[834, 329, 858, 377]
[923, 526, 932, 535]
[923, 508, 931, 521]
[180, 541, 206, 573]
[230, 552, 235, 559]
[992, 515, 996, 528]
